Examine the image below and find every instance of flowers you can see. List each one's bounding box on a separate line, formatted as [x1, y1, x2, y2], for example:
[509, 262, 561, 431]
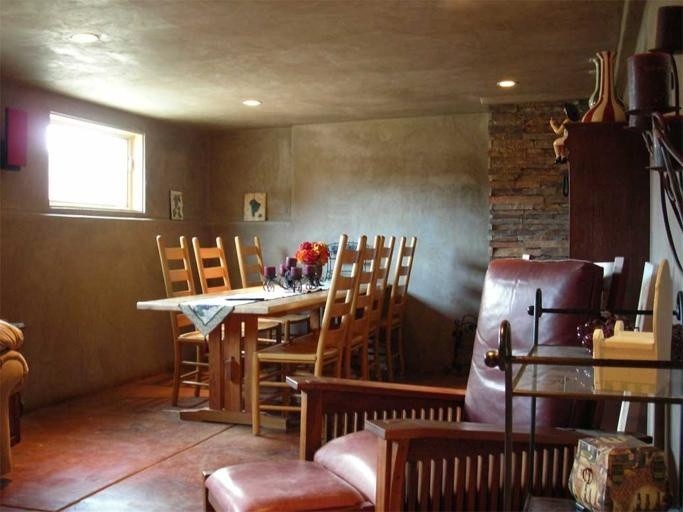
[295, 240, 329, 266]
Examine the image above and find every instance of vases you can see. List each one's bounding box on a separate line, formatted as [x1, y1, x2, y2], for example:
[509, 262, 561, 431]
[588, 57, 601, 108]
[306, 264, 323, 284]
[581, 48, 627, 123]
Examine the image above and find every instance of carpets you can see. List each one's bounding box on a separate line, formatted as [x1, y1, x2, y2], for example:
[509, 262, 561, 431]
[0, 366, 280, 512]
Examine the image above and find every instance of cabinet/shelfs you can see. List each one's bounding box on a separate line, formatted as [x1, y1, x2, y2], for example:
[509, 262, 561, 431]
[7, 322, 26, 441]
[485, 288, 683, 512]
[564, 122, 650, 431]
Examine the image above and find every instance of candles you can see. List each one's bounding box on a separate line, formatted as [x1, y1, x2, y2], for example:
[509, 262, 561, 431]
[285, 257, 297, 267]
[279, 263, 287, 273]
[290, 266, 302, 277]
[264, 265, 276, 277]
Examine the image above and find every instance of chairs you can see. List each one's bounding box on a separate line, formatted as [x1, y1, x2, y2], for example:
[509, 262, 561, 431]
[191, 235, 310, 381]
[201, 257, 655, 512]
[156, 236, 246, 409]
[284, 234, 418, 409]
[249, 234, 364, 437]
[0, 318, 29, 476]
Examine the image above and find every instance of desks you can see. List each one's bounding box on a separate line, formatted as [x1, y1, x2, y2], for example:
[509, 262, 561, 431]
[136, 283, 382, 431]
